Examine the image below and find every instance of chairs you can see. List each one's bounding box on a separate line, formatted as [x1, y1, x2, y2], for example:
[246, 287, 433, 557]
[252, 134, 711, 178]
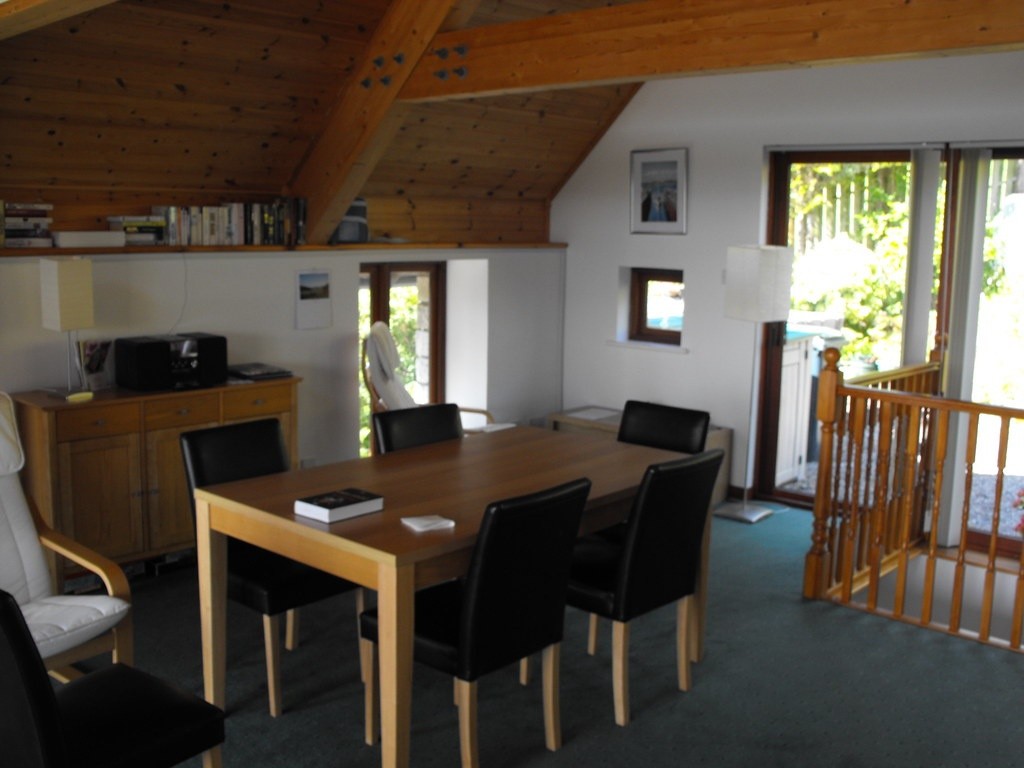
[0, 588, 228, 768]
[518, 447, 726, 727]
[0, 392, 134, 682]
[371, 402, 465, 455]
[360, 476, 592, 768]
[361, 320, 517, 455]
[617, 398, 711, 455]
[179, 417, 367, 719]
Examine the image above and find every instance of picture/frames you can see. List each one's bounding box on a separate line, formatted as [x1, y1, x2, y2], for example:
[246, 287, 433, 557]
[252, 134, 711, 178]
[628, 147, 687, 235]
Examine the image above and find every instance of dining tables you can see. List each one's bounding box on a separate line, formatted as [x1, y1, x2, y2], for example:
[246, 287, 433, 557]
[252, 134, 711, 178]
[191, 424, 711, 768]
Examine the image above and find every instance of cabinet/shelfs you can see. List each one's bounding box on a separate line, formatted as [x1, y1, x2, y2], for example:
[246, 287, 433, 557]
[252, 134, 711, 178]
[8, 374, 304, 595]
[546, 406, 736, 512]
[776, 325, 820, 490]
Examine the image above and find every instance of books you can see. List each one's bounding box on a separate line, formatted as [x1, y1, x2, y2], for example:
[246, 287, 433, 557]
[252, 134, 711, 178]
[2, 195, 308, 248]
[294, 487, 385, 524]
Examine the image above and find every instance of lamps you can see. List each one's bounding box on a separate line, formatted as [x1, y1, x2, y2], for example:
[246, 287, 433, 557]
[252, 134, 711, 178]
[713, 243, 795, 525]
